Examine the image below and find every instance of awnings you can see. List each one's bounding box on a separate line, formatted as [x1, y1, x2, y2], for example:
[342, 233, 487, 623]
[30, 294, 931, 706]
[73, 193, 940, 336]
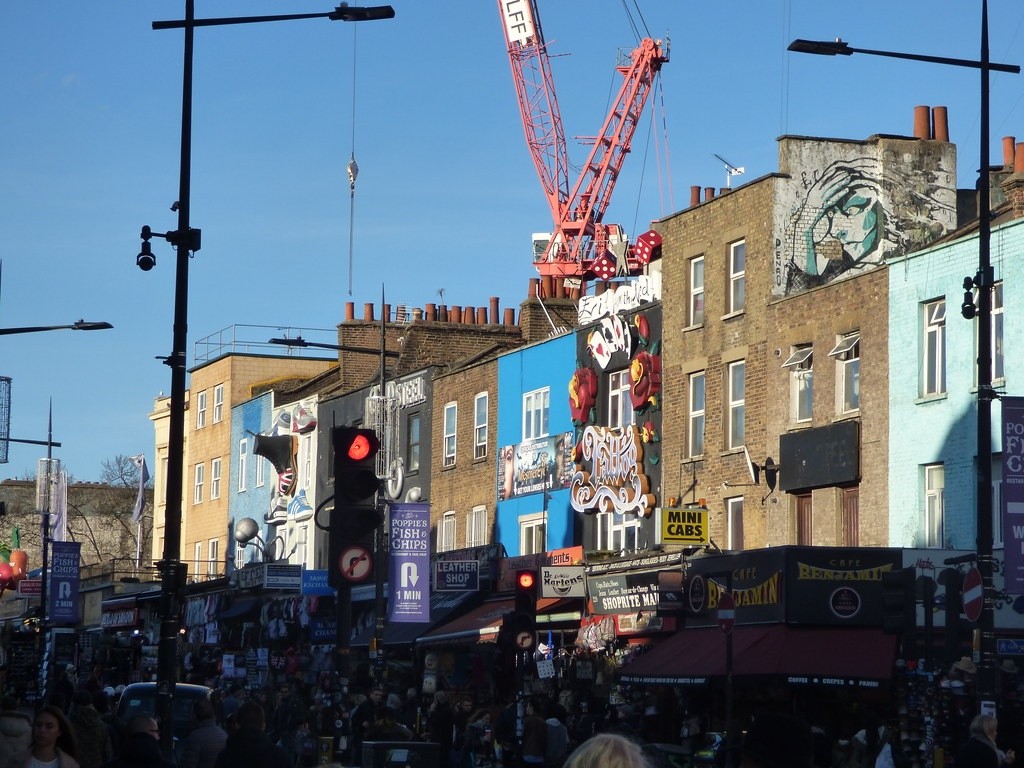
[415, 596, 577, 647]
[346, 589, 472, 647]
[715, 627, 895, 679]
[619, 627, 771, 683]
[99, 595, 163, 627]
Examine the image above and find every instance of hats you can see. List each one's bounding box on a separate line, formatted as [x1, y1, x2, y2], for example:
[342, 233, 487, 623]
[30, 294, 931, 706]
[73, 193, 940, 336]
[999, 659, 1018, 673]
[430, 690, 451, 711]
[953, 656, 977, 674]
[387, 693, 400, 709]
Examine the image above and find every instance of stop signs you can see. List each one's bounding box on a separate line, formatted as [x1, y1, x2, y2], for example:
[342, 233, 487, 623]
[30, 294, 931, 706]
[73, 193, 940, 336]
[962, 568, 983, 622]
[717, 595, 735, 633]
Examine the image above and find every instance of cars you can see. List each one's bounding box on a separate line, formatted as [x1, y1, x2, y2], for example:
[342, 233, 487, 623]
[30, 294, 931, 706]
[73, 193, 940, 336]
[0, 606, 43, 641]
[117, 681, 214, 739]
[28, 567, 51, 580]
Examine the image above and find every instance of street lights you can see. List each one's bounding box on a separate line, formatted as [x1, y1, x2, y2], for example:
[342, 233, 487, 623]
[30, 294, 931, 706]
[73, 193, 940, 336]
[788, 0, 1019, 702]
[267, 283, 400, 706]
[152, 1, 395, 768]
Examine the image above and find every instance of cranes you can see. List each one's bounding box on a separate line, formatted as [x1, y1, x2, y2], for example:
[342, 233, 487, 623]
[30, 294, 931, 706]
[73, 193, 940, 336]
[534, 36, 670, 277]
[499, 0, 573, 228]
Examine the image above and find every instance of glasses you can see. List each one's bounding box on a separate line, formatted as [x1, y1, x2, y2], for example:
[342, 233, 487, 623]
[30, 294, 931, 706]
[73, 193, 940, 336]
[463, 704, 472, 707]
[146, 729, 160, 735]
[455, 704, 461, 709]
[374, 693, 382, 697]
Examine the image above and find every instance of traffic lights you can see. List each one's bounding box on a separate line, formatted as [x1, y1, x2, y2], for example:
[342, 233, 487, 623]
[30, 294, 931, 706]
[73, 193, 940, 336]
[882, 567, 917, 639]
[330, 425, 382, 544]
[516, 569, 537, 632]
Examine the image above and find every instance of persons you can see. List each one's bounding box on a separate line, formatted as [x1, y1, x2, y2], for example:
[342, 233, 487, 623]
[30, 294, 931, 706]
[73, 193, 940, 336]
[0, 639, 1024, 768]
